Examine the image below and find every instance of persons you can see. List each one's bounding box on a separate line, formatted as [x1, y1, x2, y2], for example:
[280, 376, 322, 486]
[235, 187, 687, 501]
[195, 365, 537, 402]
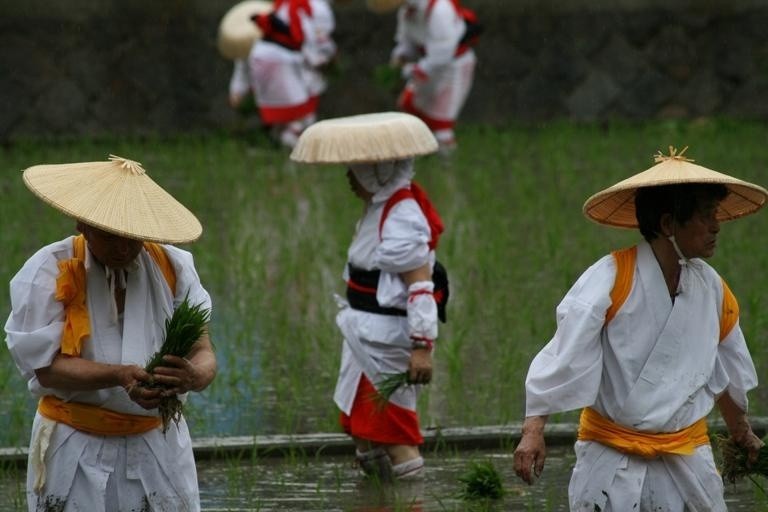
[4, 155, 216, 512]
[369, 1, 479, 173]
[513, 145, 768, 511]
[289, 110, 448, 484]
[217, 0, 337, 155]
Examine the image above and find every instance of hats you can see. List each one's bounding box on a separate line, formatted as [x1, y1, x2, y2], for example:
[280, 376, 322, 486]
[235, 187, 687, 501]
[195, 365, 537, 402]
[583, 157, 767, 228]
[23, 157, 203, 246]
[217, 0, 274, 59]
[288, 112, 439, 167]
